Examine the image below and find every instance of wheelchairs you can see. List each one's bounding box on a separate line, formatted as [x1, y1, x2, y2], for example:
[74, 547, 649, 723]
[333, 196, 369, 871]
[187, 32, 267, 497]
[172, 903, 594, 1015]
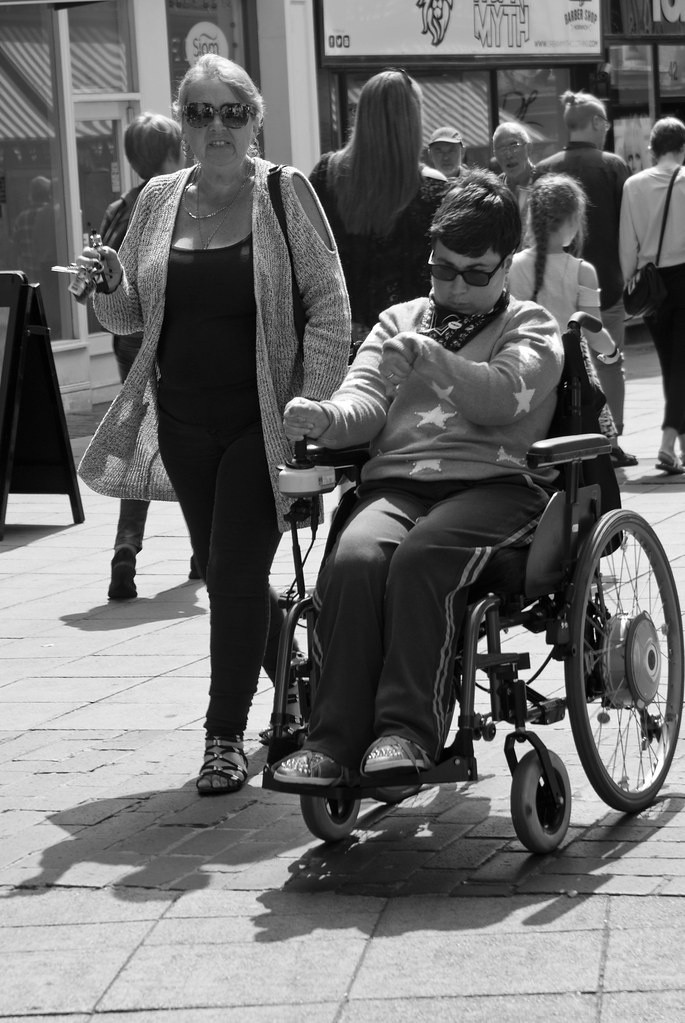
[261, 309, 685, 855]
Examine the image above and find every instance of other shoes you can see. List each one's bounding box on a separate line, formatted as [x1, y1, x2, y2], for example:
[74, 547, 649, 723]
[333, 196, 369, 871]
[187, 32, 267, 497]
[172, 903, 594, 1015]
[364, 737, 433, 769]
[610, 446, 638, 467]
[108, 550, 137, 601]
[189, 559, 199, 581]
[275, 750, 342, 785]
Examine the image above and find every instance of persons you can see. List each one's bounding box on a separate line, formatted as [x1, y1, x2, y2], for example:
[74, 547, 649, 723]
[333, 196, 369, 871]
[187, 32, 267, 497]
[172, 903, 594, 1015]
[76, 54, 352, 798]
[427, 126, 471, 181]
[493, 121, 537, 263]
[307, 67, 448, 366]
[273, 181, 565, 791]
[106, 112, 205, 601]
[620, 117, 685, 473]
[534, 88, 638, 466]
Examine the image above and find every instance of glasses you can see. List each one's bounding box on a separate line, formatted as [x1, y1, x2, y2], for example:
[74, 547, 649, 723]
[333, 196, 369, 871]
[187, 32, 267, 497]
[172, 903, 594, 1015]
[400, 69, 412, 84]
[428, 250, 505, 286]
[185, 102, 256, 129]
[496, 142, 524, 156]
[600, 116, 612, 131]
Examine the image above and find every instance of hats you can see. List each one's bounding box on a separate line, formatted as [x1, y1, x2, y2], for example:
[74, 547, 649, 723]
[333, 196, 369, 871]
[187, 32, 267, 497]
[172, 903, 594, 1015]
[429, 126, 463, 144]
[28, 175, 54, 202]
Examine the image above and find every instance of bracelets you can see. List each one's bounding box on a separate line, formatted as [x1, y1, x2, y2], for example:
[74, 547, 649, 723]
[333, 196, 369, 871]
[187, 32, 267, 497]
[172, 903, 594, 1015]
[606, 343, 618, 358]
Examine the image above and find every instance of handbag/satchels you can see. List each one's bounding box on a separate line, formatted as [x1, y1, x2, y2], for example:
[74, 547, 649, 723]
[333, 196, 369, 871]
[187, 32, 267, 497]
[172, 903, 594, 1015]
[624, 263, 666, 317]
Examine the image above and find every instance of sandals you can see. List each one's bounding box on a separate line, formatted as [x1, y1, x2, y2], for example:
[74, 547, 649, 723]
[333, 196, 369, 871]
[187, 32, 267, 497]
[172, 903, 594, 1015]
[656, 450, 685, 473]
[258, 679, 302, 745]
[197, 735, 249, 797]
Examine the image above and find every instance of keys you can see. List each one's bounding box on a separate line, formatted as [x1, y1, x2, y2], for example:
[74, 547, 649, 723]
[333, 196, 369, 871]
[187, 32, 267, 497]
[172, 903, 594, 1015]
[51, 257, 111, 302]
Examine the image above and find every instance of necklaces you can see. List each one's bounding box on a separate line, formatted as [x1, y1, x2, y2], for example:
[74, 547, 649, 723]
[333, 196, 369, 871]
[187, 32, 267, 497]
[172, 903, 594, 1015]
[181, 162, 255, 220]
